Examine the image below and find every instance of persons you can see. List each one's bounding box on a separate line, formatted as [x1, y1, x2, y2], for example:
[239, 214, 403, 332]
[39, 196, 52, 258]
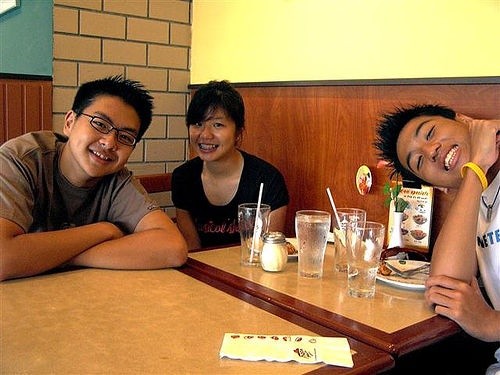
[374, 104, 500, 375]
[172, 80, 288, 251]
[0, 73, 189, 282]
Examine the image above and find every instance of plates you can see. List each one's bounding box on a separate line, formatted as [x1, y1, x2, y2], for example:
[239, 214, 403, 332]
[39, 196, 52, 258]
[367, 259, 432, 290]
[247, 237, 298, 260]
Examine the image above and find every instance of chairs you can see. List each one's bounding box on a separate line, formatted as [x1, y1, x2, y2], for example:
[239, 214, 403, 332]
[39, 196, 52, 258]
[133, 173, 176, 224]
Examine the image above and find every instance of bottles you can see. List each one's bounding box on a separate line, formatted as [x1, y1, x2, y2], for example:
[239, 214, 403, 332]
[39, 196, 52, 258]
[260, 231, 288, 272]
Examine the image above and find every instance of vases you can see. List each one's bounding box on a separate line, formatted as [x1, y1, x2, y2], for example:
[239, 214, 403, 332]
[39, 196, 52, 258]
[387, 211, 405, 249]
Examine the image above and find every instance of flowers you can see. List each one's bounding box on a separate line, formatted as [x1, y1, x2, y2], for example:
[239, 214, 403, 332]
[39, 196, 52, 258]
[377, 156, 409, 212]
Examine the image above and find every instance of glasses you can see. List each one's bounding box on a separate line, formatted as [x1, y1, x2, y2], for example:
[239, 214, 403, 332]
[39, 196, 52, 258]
[80, 110, 137, 147]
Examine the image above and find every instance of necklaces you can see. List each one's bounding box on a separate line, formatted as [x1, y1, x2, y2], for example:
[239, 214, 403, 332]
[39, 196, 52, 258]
[482, 186, 500, 222]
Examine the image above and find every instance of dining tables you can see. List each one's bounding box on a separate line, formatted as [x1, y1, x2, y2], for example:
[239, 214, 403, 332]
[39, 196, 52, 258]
[0, 231, 495, 375]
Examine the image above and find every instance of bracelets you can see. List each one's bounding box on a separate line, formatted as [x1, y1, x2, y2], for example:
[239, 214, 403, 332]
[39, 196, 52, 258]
[460, 162, 487, 192]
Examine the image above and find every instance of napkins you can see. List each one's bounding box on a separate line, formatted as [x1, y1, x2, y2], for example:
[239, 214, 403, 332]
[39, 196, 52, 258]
[326, 231, 335, 244]
[219, 332, 354, 369]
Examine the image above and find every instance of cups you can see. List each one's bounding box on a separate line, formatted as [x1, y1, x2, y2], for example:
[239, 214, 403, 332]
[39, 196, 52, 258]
[294, 209, 331, 280]
[333, 208, 366, 272]
[345, 220, 385, 297]
[238, 203, 271, 268]
